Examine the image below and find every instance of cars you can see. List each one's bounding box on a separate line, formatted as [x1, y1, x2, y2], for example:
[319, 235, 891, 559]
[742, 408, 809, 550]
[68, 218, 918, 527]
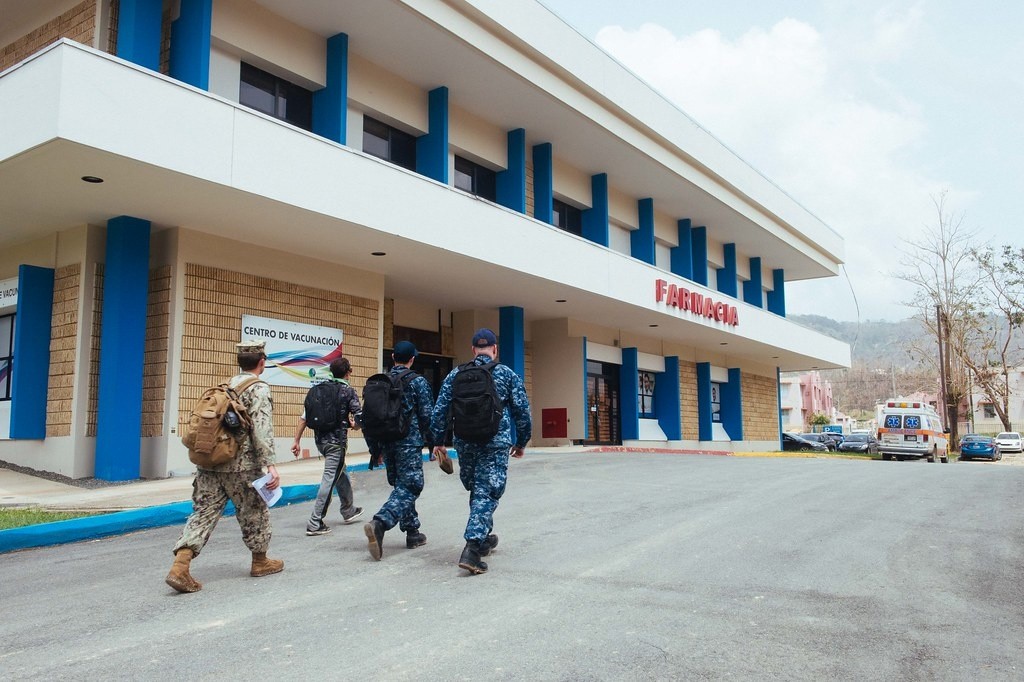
[802, 433, 845, 450]
[961, 435, 1002, 461]
[781, 432, 829, 453]
[852, 430, 873, 434]
[995, 432, 1024, 453]
[839, 433, 878, 454]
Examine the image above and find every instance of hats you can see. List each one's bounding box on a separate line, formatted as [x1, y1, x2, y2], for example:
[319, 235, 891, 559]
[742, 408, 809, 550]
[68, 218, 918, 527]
[236, 339, 268, 357]
[393, 341, 419, 358]
[473, 328, 497, 347]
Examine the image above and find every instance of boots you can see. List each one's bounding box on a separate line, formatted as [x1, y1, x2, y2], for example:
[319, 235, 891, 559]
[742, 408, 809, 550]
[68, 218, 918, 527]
[458, 539, 488, 574]
[251, 552, 284, 577]
[165, 548, 202, 592]
[478, 535, 498, 556]
[364, 518, 386, 560]
[406, 527, 426, 548]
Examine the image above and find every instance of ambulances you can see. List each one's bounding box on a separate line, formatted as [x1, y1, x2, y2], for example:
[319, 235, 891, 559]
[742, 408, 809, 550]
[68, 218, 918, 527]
[877, 400, 950, 463]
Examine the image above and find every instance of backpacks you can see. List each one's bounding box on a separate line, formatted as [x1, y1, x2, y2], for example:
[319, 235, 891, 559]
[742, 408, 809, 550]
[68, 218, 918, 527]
[362, 370, 418, 442]
[448, 361, 510, 443]
[182, 376, 269, 467]
[304, 380, 350, 431]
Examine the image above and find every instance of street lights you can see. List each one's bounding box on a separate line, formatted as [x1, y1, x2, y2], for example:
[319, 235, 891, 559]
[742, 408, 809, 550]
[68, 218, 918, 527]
[934, 302, 947, 429]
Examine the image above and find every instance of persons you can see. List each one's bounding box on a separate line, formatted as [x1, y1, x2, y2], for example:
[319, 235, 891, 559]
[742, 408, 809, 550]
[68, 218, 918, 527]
[432, 328, 532, 574]
[291, 358, 364, 536]
[364, 341, 435, 562]
[165, 339, 285, 594]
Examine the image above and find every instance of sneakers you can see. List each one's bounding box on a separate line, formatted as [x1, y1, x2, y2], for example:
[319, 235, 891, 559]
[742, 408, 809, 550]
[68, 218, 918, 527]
[344, 507, 364, 522]
[306, 524, 331, 536]
